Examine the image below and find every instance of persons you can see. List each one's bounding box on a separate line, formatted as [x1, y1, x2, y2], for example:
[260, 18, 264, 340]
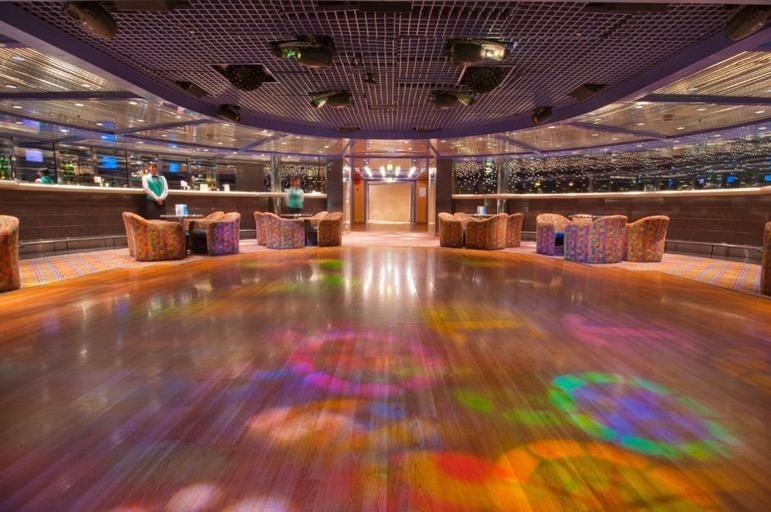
[141, 160, 169, 218]
[287, 178, 305, 213]
[34, 167, 55, 184]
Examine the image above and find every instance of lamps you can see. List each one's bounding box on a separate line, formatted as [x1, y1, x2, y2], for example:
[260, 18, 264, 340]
[216, 105, 240, 123]
[723, 5, 771, 43]
[434, 92, 458, 109]
[295, 47, 334, 68]
[453, 42, 486, 64]
[326, 93, 352, 109]
[178, 81, 205, 99]
[62, 0, 117, 40]
[531, 106, 554, 125]
[567, 83, 607, 102]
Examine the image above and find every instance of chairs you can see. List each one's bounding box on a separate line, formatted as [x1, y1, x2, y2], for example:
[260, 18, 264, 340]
[253, 210, 343, 249]
[0, 214, 20, 293]
[121, 210, 243, 262]
[535, 212, 670, 263]
[437, 211, 525, 250]
